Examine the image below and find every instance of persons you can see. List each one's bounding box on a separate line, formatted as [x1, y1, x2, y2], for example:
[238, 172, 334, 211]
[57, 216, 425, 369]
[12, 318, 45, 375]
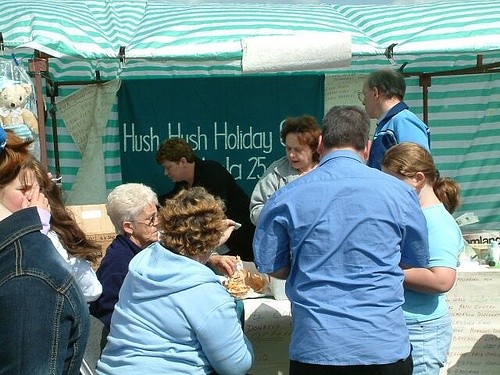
[359, 71, 431, 173]
[0, 127, 102, 375]
[381, 142, 464, 375]
[252, 104, 429, 375]
[248, 114, 321, 226]
[94, 186, 255, 375]
[89, 183, 237, 329]
[156, 138, 256, 262]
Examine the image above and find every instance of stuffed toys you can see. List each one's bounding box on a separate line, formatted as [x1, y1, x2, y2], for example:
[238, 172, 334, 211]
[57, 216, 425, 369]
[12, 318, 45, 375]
[0, 80, 39, 151]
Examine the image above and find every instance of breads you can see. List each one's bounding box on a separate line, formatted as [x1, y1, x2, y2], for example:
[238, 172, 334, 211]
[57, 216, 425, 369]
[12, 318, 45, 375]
[222, 269, 265, 297]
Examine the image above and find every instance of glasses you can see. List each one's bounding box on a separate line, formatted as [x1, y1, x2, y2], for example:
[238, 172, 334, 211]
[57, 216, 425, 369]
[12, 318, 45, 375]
[131, 210, 161, 227]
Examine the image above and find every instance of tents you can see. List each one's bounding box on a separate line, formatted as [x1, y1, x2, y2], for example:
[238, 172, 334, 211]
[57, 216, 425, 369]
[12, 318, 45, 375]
[0, 0, 500, 231]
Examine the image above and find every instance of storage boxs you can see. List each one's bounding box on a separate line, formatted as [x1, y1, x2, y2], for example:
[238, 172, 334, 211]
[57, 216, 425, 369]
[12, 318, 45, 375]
[66, 203, 118, 269]
